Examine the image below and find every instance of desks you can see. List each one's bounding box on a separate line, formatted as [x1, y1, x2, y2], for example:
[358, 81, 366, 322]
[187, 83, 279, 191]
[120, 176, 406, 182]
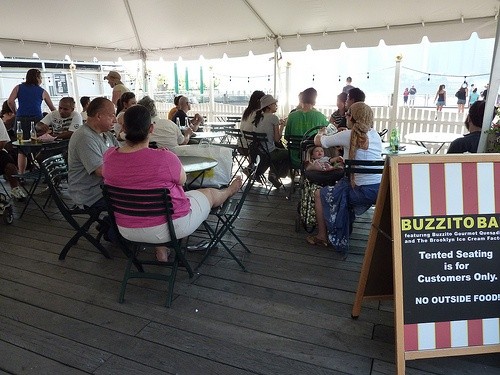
[178, 156, 219, 193]
[190, 132, 228, 144]
[405, 132, 465, 155]
[12, 138, 68, 220]
[210, 122, 236, 132]
[380, 143, 427, 155]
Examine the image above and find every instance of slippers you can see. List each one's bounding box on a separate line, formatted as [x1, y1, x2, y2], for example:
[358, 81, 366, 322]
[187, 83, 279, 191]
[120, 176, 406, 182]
[187, 239, 218, 251]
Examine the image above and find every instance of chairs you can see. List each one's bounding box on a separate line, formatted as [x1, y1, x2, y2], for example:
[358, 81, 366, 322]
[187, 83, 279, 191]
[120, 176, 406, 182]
[0, 116, 393, 307]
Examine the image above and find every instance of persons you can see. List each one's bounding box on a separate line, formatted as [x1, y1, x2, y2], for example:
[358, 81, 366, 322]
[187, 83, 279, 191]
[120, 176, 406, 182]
[403, 85, 417, 106]
[455, 83, 500, 112]
[237, 77, 386, 250]
[434, 85, 446, 120]
[0, 68, 202, 241]
[101, 105, 243, 262]
[448, 101, 496, 155]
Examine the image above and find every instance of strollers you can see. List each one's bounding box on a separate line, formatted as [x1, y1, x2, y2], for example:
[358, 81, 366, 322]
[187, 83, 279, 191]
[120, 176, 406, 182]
[0, 148, 24, 224]
[296, 125, 345, 232]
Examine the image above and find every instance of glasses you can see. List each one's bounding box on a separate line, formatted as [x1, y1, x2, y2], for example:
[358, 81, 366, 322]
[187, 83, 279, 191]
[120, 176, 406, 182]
[345, 112, 350, 116]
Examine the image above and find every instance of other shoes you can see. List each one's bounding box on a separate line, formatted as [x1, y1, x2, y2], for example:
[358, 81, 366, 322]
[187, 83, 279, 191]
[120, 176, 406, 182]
[243, 166, 255, 180]
[19, 177, 24, 183]
[307, 235, 328, 248]
[11, 186, 25, 199]
[40, 186, 51, 198]
[268, 173, 281, 188]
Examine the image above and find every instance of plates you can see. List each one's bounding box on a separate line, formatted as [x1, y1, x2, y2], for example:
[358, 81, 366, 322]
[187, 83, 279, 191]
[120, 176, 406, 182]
[32, 137, 57, 142]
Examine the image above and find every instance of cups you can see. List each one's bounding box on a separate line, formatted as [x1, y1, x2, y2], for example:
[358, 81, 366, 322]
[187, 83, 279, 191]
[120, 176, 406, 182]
[325, 123, 337, 136]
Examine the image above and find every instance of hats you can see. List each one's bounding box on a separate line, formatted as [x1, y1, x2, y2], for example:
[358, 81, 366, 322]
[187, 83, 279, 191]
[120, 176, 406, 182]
[259, 94, 278, 111]
[350, 102, 374, 127]
[109, 70, 121, 80]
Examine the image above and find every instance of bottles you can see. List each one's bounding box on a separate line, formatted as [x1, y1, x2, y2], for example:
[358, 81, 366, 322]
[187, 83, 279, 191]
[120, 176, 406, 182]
[176, 117, 181, 129]
[17, 121, 23, 145]
[30, 121, 37, 144]
[390, 129, 398, 153]
[184, 117, 190, 129]
